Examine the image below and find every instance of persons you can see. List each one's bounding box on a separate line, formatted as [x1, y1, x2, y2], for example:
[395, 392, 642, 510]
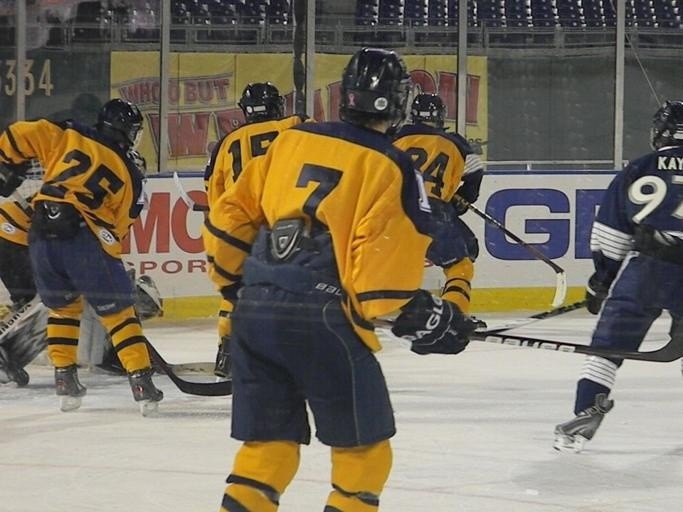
[557, 100, 683, 448]
[202, 49, 466, 511]
[204, 83, 320, 381]
[392, 94, 485, 314]
[1, 98, 164, 417]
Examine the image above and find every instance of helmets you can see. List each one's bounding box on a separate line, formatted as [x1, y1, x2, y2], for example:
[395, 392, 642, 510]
[338, 49, 412, 117]
[96, 100, 144, 152]
[650, 100, 683, 152]
[413, 93, 448, 122]
[237, 82, 285, 123]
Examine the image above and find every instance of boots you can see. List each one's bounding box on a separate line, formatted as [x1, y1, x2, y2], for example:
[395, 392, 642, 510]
[214, 334, 235, 379]
[554, 393, 614, 440]
[0, 361, 163, 402]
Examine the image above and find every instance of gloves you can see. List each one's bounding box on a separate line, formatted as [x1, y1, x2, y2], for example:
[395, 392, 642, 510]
[449, 186, 479, 216]
[390, 290, 472, 354]
[0, 162, 31, 197]
[585, 270, 616, 315]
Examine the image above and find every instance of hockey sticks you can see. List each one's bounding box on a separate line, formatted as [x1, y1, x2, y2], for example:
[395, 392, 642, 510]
[173, 168, 209, 213]
[374, 310, 683, 365]
[458, 194, 567, 308]
[143, 334, 232, 397]
[468, 299, 593, 336]
